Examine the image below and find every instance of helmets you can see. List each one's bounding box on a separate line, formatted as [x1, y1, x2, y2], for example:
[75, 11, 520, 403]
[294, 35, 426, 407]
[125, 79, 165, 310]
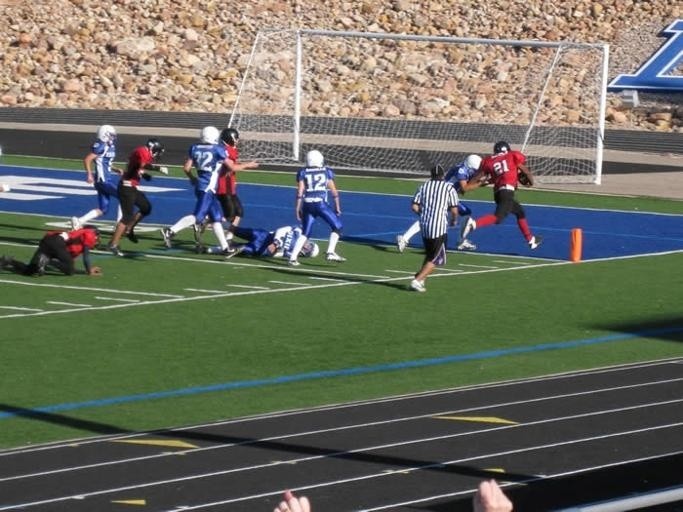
[494, 142, 509, 152]
[146, 139, 164, 160]
[219, 129, 239, 149]
[306, 150, 323, 168]
[464, 155, 483, 171]
[97, 124, 116, 144]
[201, 126, 219, 145]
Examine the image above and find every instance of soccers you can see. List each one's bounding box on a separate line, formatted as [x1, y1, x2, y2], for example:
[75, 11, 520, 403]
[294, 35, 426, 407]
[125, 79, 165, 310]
[518, 172, 529, 185]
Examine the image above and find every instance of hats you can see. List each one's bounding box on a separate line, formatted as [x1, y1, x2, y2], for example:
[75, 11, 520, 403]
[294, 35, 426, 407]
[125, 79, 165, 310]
[432, 164, 444, 176]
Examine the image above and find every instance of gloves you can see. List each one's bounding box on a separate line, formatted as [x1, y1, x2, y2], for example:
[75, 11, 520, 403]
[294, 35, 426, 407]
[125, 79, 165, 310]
[478, 172, 488, 184]
[159, 167, 169, 175]
[142, 173, 150, 181]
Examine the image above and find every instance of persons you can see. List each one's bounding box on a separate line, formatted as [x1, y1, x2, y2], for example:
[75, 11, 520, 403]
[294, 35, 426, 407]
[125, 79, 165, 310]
[396, 154, 483, 251]
[288, 149, 347, 266]
[411, 164, 460, 291]
[160, 126, 240, 259]
[71, 124, 124, 232]
[193, 128, 260, 246]
[108, 139, 168, 257]
[273, 479, 514, 512]
[1, 224, 104, 278]
[461, 140, 543, 250]
[195, 222, 320, 258]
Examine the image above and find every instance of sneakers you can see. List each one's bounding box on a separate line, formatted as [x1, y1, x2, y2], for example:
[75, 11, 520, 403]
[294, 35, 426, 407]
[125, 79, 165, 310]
[529, 236, 543, 250]
[224, 246, 244, 258]
[105, 241, 126, 257]
[160, 227, 175, 248]
[38, 253, 50, 274]
[461, 217, 473, 238]
[71, 216, 82, 231]
[288, 260, 300, 266]
[123, 229, 138, 244]
[194, 219, 208, 255]
[326, 253, 346, 262]
[457, 242, 476, 251]
[0, 255, 11, 264]
[396, 235, 407, 252]
[411, 273, 426, 292]
